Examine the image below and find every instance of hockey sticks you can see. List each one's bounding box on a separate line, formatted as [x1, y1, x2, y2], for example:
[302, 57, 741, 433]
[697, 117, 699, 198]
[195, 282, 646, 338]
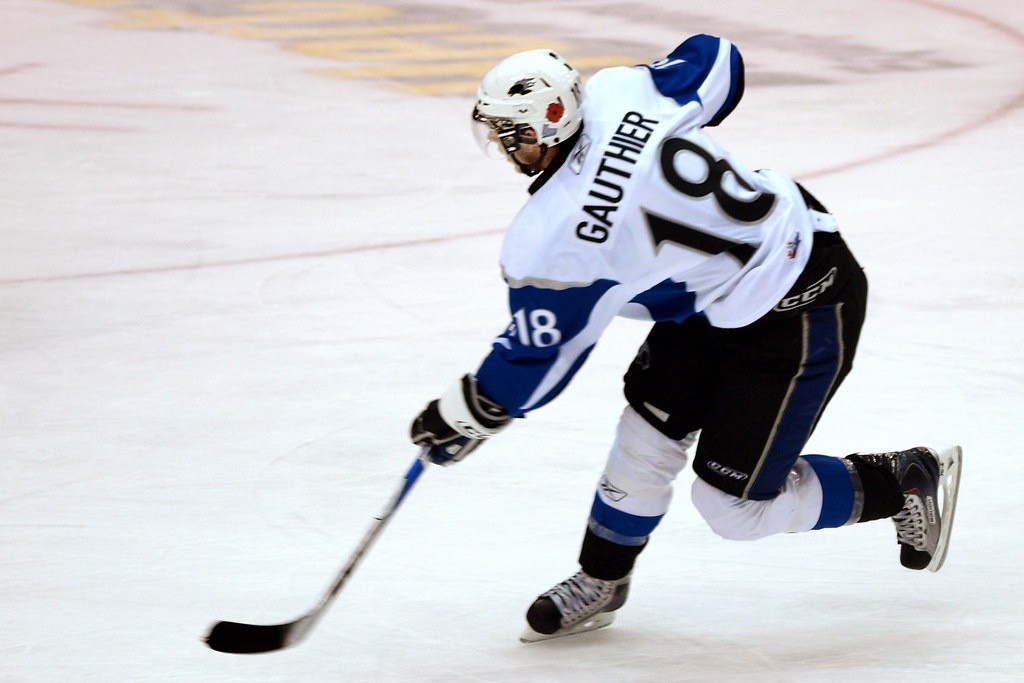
[200, 440, 437, 656]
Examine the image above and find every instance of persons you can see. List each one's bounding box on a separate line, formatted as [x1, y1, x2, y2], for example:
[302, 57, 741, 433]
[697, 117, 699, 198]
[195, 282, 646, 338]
[410, 33, 965, 643]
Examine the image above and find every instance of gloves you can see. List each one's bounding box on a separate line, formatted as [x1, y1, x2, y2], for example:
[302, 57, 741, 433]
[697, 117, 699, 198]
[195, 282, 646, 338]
[411, 372, 509, 466]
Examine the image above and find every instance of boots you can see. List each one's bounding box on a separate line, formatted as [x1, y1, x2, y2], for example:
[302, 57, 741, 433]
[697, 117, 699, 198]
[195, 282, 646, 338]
[520, 526, 649, 642]
[847, 446, 963, 569]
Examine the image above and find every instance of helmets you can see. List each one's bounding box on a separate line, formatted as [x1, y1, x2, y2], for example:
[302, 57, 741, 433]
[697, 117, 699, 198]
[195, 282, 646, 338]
[473, 48, 585, 147]
